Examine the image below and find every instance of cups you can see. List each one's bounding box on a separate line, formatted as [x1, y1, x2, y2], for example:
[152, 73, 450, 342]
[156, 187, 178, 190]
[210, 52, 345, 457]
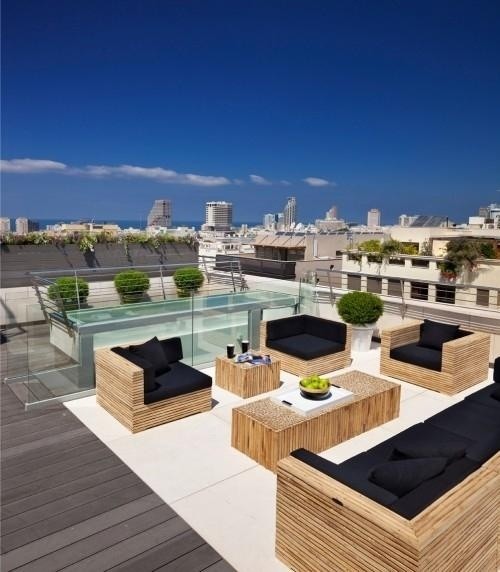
[227, 344, 235, 359]
[241, 340, 249, 353]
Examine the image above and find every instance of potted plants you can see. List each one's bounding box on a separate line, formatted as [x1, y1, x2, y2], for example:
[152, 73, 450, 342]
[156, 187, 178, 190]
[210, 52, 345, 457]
[336, 289, 384, 351]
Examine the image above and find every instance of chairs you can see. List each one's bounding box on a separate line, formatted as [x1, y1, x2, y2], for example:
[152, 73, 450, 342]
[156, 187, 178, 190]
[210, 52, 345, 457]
[129, 337, 169, 375]
[379, 321, 491, 396]
[259, 313, 352, 379]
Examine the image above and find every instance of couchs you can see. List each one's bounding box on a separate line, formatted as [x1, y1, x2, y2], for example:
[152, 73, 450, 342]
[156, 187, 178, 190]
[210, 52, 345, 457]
[93, 340, 213, 434]
[275, 358, 500, 572]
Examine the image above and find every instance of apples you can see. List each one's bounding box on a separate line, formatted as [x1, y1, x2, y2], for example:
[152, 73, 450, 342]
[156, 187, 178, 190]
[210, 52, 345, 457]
[301, 376, 329, 389]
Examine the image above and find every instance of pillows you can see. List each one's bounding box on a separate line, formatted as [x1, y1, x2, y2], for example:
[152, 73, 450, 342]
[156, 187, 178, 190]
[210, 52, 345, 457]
[416, 319, 459, 352]
[368, 439, 467, 493]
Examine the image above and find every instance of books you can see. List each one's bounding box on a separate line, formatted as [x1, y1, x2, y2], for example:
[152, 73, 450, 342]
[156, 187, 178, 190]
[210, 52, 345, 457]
[234, 352, 272, 365]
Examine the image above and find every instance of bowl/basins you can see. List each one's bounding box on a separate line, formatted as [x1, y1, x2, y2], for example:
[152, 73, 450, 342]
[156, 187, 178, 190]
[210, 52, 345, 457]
[299, 381, 331, 397]
